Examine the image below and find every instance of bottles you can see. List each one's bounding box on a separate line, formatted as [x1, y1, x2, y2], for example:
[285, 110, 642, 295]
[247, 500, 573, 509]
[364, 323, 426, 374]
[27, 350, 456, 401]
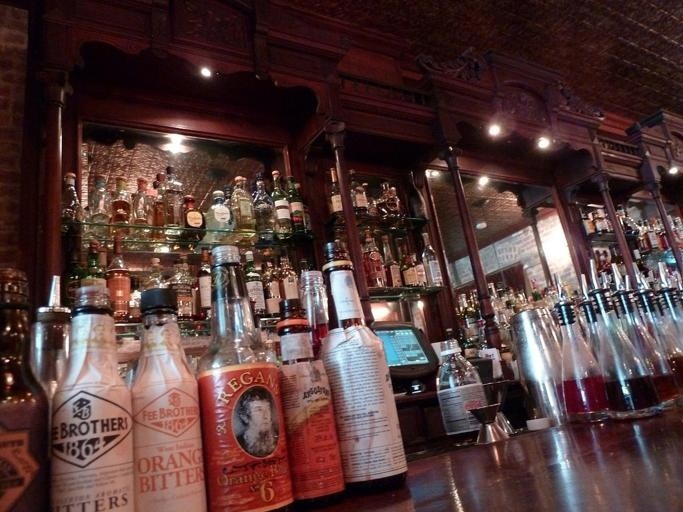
[29, 274, 72, 398]
[4, 169, 441, 327]
[322, 236, 409, 495]
[4, 330, 53, 508]
[461, 204, 683, 427]
[45, 285, 134, 508]
[274, 296, 348, 506]
[431, 339, 493, 447]
[128, 288, 208, 510]
[191, 245, 299, 507]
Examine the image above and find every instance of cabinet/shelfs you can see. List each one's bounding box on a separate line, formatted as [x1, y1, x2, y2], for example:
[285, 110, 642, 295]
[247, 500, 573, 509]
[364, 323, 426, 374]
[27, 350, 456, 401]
[543, 75, 683, 298]
[22, 1, 459, 460]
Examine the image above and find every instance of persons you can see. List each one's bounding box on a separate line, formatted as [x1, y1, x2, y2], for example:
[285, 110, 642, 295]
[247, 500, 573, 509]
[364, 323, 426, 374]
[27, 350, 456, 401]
[236, 387, 278, 460]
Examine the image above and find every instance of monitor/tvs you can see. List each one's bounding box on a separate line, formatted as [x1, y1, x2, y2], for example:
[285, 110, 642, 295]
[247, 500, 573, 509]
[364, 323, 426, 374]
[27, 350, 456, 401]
[371, 321, 439, 382]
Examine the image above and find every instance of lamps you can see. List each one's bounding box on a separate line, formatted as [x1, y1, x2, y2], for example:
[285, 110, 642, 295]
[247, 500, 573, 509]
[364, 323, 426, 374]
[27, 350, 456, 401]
[665, 147, 678, 175]
